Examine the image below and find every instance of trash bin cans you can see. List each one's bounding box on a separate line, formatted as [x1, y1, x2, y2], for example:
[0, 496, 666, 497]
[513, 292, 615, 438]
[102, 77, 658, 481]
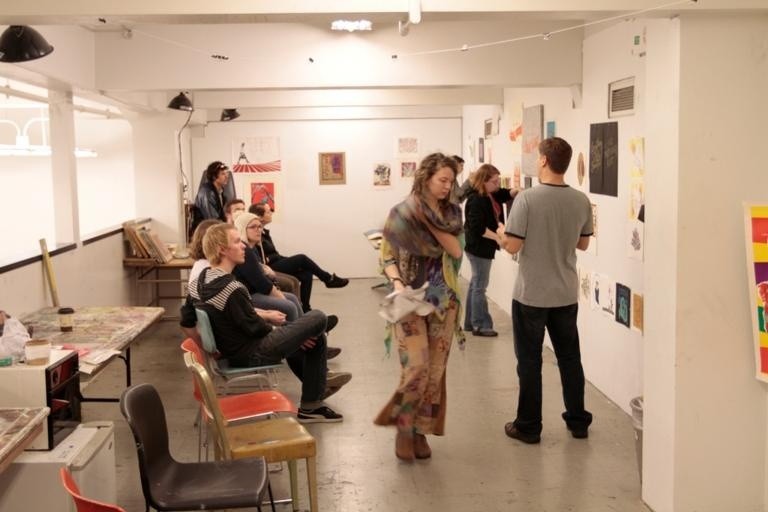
[630, 396, 643, 485]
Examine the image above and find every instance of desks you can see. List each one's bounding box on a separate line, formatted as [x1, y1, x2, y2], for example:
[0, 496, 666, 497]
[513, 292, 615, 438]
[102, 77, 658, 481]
[19, 309, 164, 404]
[0, 405, 52, 474]
[122, 256, 197, 306]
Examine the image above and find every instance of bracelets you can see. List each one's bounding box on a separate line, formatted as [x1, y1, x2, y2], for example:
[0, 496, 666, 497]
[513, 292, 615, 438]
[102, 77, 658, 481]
[390, 278, 406, 287]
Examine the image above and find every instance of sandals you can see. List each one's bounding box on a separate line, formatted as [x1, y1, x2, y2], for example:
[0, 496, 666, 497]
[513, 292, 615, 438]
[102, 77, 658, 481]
[472, 325, 498, 336]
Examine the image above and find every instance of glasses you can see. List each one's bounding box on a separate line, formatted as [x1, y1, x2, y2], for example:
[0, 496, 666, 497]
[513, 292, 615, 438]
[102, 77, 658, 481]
[247, 223, 263, 233]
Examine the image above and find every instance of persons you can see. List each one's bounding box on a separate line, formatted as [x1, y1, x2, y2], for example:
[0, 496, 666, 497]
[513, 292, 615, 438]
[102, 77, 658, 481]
[178, 162, 228, 243]
[248, 203, 349, 313]
[447, 155, 473, 207]
[232, 210, 341, 360]
[496, 137, 592, 443]
[180, 224, 344, 422]
[187, 218, 352, 400]
[223, 200, 304, 306]
[464, 164, 519, 336]
[380, 152, 467, 461]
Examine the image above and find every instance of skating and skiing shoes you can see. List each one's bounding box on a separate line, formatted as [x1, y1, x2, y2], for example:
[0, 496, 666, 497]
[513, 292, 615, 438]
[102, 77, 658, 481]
[325, 370, 353, 388]
[296, 406, 343, 425]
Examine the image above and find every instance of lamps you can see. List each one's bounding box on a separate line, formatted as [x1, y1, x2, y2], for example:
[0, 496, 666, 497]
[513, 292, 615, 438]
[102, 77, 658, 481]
[221, 108, 239, 123]
[1, 25, 53, 63]
[166, 92, 194, 111]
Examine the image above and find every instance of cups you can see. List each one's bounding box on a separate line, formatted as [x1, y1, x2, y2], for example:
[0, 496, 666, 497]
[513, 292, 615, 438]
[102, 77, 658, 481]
[57, 307, 74, 332]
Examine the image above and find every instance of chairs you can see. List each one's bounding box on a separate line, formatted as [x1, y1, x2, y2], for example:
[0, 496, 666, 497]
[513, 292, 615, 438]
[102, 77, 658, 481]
[177, 337, 297, 430]
[195, 311, 287, 426]
[182, 352, 319, 510]
[118, 382, 275, 509]
[58, 468, 129, 511]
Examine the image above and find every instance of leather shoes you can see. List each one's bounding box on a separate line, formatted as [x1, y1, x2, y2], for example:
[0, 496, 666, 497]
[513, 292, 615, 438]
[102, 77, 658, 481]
[505, 421, 541, 444]
[562, 409, 588, 438]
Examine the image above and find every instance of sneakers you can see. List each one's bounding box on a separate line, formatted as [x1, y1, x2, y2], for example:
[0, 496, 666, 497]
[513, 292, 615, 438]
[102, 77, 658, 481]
[395, 430, 416, 460]
[324, 315, 338, 332]
[325, 273, 349, 288]
[325, 346, 341, 360]
[412, 428, 431, 459]
